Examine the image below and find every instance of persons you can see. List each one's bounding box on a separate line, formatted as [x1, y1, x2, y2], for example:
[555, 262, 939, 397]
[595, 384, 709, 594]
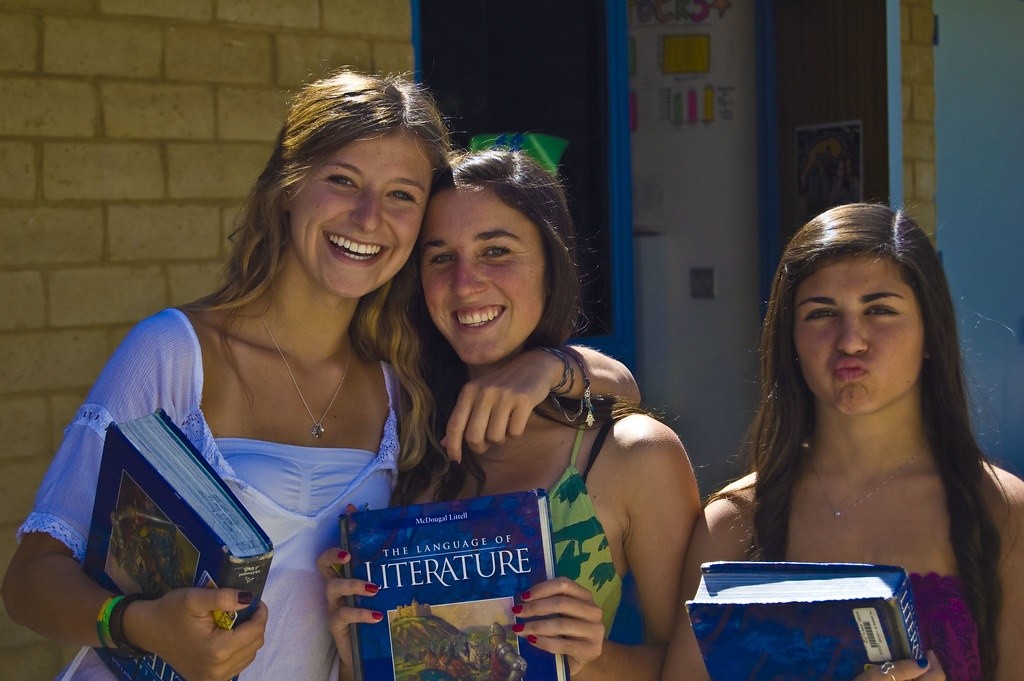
[0, 64, 640, 681]
[315, 147, 703, 681]
[660, 204, 1024, 681]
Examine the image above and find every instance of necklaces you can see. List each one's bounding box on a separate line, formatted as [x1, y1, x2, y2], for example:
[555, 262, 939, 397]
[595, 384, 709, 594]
[808, 444, 929, 517]
[252, 300, 351, 439]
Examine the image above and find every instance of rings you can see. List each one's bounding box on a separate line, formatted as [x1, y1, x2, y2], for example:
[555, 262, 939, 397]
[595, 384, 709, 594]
[879, 662, 899, 681]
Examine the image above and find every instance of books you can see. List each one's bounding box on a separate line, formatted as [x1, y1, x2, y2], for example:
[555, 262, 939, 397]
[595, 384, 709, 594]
[338, 488, 571, 681]
[82, 408, 274, 681]
[685, 561, 926, 681]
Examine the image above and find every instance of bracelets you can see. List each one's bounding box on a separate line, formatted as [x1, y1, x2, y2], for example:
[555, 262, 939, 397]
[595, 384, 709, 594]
[523, 343, 597, 427]
[96, 592, 157, 660]
